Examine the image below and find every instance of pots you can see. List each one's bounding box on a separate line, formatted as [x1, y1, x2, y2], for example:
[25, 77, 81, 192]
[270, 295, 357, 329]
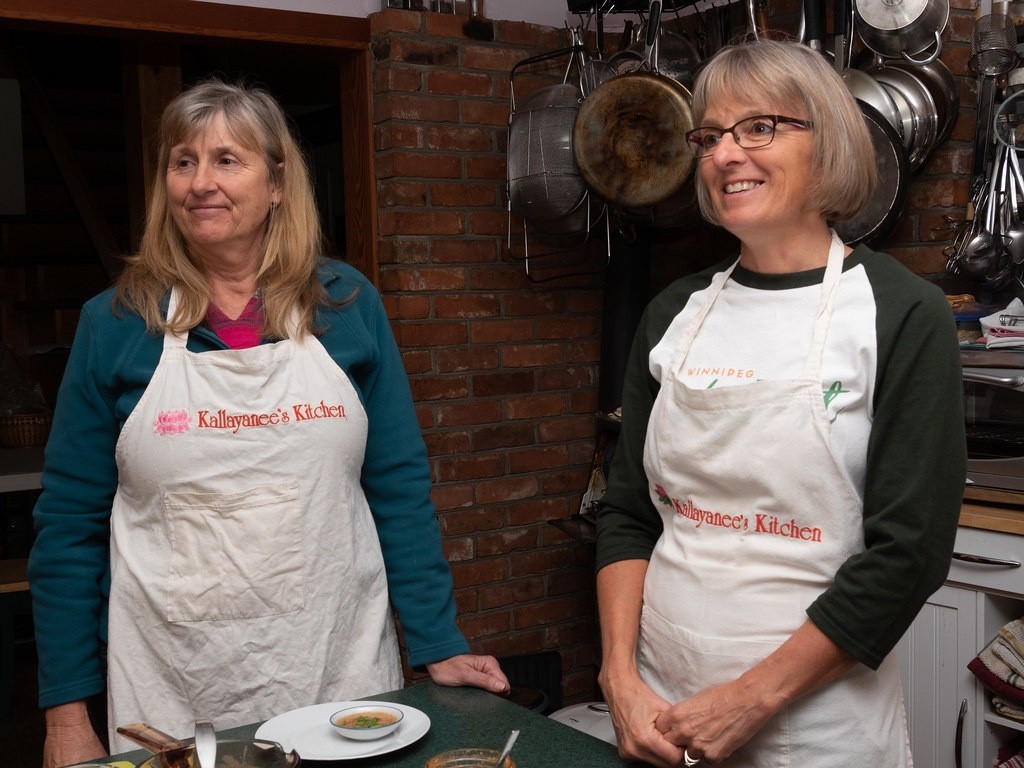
[116, 721, 301, 768]
[569, 0, 960, 247]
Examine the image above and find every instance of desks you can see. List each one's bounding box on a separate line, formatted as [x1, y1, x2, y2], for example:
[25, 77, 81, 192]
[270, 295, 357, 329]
[46, 678, 664, 768]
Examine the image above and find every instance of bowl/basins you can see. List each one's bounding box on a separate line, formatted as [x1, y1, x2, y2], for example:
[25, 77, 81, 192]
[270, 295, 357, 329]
[329, 705, 404, 741]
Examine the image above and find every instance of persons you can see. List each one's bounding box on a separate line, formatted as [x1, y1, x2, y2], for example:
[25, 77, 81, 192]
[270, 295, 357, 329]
[24, 82, 511, 768]
[592, 35, 966, 768]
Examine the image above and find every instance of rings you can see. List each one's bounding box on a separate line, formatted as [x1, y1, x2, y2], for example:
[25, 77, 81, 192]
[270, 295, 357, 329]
[683, 749, 700, 768]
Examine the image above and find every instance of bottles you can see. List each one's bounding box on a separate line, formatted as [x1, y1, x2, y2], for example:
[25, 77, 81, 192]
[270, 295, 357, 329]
[425, 746, 516, 768]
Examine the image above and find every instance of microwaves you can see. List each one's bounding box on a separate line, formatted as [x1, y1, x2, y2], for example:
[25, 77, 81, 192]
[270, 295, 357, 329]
[959, 344, 1024, 507]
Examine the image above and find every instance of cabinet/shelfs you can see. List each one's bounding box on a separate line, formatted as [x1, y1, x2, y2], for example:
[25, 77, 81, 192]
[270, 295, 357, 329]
[894, 527, 1024, 768]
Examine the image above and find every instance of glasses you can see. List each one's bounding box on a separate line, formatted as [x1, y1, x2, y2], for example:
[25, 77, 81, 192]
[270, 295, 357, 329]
[686, 114, 814, 159]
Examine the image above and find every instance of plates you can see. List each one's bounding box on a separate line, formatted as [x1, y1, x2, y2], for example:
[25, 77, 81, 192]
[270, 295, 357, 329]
[254, 700, 431, 761]
